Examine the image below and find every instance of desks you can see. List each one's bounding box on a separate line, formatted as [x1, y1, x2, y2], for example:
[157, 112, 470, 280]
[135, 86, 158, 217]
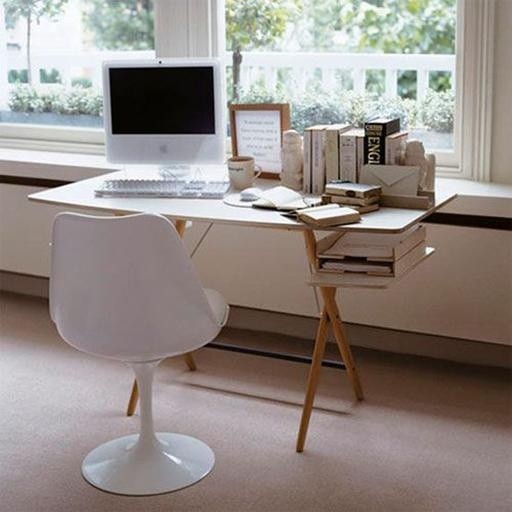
[24, 173, 459, 454]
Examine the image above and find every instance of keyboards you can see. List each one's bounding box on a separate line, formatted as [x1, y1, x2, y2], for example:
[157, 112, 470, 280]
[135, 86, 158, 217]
[92, 178, 232, 200]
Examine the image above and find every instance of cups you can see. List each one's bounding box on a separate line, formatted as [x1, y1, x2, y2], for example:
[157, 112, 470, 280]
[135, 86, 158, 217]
[227, 155, 261, 190]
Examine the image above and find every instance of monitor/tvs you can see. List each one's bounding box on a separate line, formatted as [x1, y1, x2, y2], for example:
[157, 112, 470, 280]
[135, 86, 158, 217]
[100, 58, 227, 166]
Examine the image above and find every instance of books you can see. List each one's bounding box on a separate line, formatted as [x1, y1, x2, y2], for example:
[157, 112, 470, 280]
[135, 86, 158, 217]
[252, 118, 426, 278]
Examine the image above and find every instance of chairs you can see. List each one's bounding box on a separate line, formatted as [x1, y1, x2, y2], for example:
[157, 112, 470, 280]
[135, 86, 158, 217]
[46, 209, 230, 496]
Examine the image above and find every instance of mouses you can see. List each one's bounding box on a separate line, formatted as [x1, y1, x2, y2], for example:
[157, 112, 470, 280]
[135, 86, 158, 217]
[241, 187, 262, 202]
